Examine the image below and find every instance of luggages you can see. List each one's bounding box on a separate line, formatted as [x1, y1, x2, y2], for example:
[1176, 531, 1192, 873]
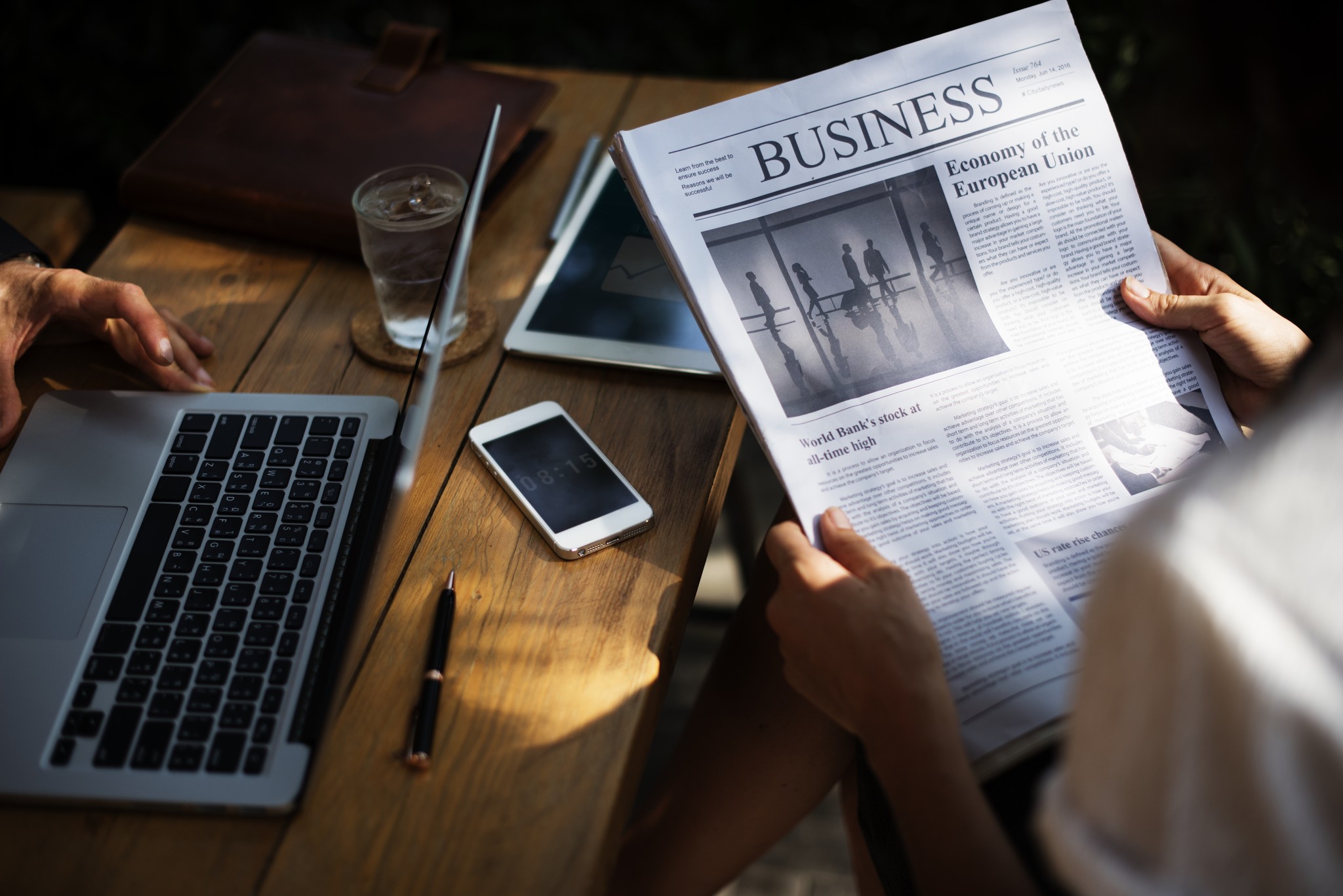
[841, 291, 857, 312]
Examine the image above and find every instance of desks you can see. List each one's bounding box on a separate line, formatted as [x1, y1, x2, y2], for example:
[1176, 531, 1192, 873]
[9, 54, 752, 895]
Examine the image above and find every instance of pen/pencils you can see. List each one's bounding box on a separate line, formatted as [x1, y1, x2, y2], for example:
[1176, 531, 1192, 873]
[402, 568, 460, 770]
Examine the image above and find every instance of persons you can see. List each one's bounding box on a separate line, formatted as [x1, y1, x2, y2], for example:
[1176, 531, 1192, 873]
[792, 263, 828, 319]
[769, 156, 1343, 896]
[842, 244, 879, 309]
[0, 216, 215, 439]
[920, 222, 954, 286]
[746, 271, 781, 334]
[863, 239, 900, 300]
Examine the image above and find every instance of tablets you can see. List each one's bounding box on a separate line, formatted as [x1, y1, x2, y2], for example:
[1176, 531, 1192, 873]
[502, 148, 723, 376]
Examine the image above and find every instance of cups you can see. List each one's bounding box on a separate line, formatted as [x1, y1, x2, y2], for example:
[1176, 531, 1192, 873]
[353, 165, 468, 348]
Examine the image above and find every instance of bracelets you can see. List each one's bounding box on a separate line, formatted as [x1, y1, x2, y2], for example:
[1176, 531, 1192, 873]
[22, 255, 42, 268]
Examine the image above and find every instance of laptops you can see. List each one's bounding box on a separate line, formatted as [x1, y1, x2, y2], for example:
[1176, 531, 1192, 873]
[0, 99, 509, 813]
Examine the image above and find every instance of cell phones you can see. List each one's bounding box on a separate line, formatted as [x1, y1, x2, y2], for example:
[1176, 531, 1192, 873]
[467, 395, 656, 561]
